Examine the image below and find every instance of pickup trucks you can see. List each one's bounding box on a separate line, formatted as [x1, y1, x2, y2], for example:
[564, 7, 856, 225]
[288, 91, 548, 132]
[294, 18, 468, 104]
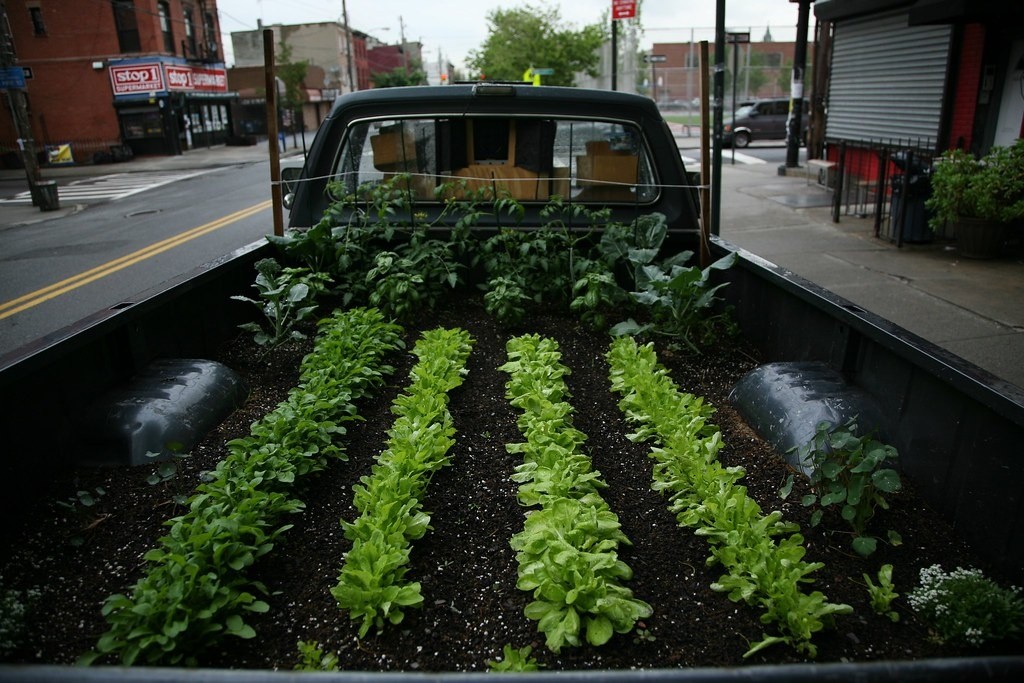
[3, 81, 1024, 682]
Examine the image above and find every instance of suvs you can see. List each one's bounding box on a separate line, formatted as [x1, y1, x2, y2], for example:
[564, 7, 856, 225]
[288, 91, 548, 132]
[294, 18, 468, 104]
[720, 96, 812, 151]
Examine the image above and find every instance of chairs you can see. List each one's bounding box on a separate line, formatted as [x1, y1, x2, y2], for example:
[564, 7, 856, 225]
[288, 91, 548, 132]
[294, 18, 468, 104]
[445, 166, 546, 200]
[385, 174, 434, 199]
[552, 165, 624, 203]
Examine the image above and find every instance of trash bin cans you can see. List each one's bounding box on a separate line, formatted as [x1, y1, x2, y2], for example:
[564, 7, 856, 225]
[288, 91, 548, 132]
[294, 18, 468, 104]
[30, 179, 61, 212]
[884, 147, 943, 247]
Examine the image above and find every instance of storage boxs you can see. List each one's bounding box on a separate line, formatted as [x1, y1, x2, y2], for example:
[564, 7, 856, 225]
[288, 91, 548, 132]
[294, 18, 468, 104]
[371, 134, 418, 174]
[576, 142, 638, 188]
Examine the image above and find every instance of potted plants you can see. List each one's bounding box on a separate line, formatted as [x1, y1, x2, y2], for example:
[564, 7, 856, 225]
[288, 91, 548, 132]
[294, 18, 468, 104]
[924, 137, 1024, 260]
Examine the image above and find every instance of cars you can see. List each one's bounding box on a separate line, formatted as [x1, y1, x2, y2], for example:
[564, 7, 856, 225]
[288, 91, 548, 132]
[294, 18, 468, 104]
[653, 94, 761, 113]
